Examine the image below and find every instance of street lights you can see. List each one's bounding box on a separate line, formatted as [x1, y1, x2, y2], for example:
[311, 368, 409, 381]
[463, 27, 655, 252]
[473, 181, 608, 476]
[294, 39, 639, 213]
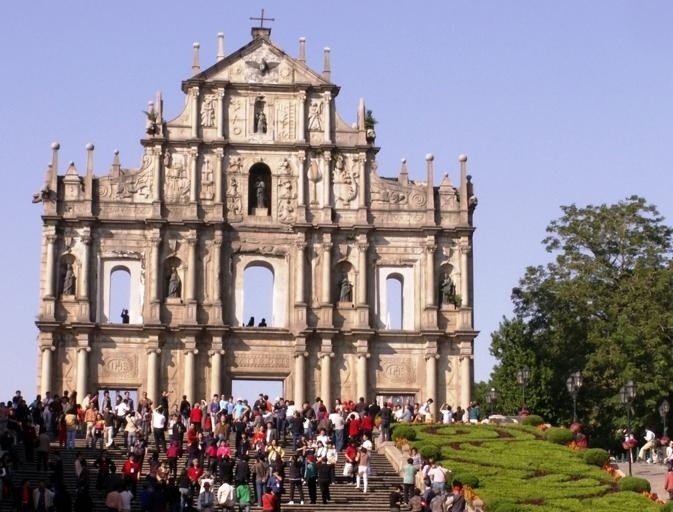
[659, 400, 671, 435]
[516, 366, 531, 404]
[620, 380, 638, 476]
[485, 386, 498, 414]
[565, 369, 584, 423]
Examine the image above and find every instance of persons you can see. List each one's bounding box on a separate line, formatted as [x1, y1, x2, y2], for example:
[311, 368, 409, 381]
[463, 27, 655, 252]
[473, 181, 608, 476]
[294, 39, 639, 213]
[165, 266, 180, 297]
[364, 111, 376, 130]
[226, 176, 240, 195]
[338, 271, 352, 301]
[439, 272, 455, 305]
[252, 177, 265, 216]
[305, 101, 322, 130]
[664, 464, 672, 499]
[119, 309, 129, 323]
[199, 101, 214, 129]
[276, 179, 293, 198]
[61, 262, 74, 294]
[0, 391, 487, 512]
[254, 109, 266, 133]
[225, 156, 243, 174]
[271, 157, 291, 176]
[245, 316, 254, 328]
[258, 319, 266, 326]
[615, 427, 672, 472]
